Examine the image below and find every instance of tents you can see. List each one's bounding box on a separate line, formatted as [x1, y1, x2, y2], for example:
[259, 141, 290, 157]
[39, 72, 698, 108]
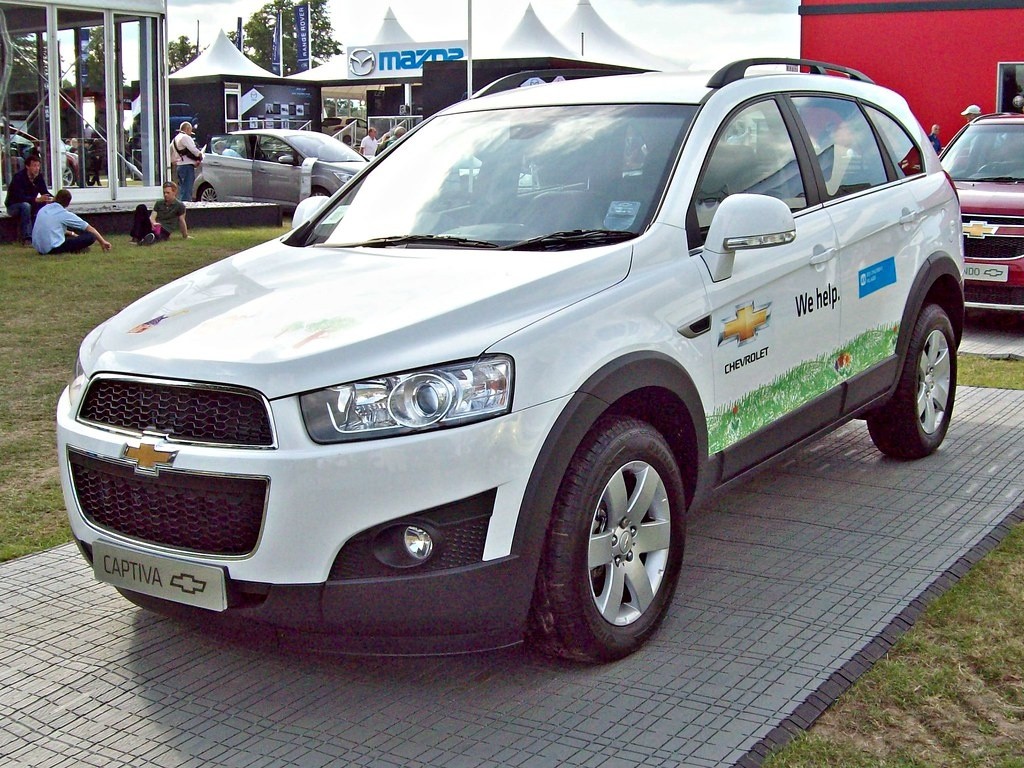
[130, 0, 664, 182]
[0, 86, 130, 175]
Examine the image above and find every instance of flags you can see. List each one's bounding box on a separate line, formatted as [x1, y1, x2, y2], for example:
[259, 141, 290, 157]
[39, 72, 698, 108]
[293, 5, 309, 72]
[79, 28, 90, 86]
[271, 11, 280, 75]
[237, 17, 243, 54]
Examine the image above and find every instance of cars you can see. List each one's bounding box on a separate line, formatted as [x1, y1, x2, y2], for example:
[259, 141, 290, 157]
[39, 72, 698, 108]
[170, 103, 201, 137]
[459, 155, 532, 196]
[0, 123, 80, 187]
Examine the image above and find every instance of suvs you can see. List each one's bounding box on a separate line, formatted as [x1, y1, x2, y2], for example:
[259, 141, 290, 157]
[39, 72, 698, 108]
[323, 115, 367, 146]
[55, 56, 965, 665]
[194, 129, 372, 207]
[937, 111, 1024, 311]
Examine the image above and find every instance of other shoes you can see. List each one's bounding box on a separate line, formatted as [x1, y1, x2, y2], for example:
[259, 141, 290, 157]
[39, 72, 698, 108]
[23, 240, 31, 247]
[141, 233, 155, 245]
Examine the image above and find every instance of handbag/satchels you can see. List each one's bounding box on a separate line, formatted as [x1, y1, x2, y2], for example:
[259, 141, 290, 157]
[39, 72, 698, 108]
[185, 148, 200, 159]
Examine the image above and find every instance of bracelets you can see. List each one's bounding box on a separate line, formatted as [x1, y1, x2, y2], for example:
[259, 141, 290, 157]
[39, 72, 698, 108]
[71, 231, 74, 236]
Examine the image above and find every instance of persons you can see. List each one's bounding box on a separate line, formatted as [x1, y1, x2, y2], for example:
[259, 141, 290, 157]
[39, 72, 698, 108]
[129, 181, 194, 246]
[32, 189, 112, 254]
[83, 124, 92, 138]
[169, 122, 201, 204]
[359, 127, 377, 155]
[4, 155, 55, 248]
[961, 104, 981, 122]
[386, 127, 407, 147]
[375, 135, 390, 157]
[214, 141, 243, 157]
[65, 133, 143, 186]
[928, 124, 942, 158]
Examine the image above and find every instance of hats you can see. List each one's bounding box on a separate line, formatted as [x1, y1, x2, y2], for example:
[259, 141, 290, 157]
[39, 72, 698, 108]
[961, 105, 981, 115]
